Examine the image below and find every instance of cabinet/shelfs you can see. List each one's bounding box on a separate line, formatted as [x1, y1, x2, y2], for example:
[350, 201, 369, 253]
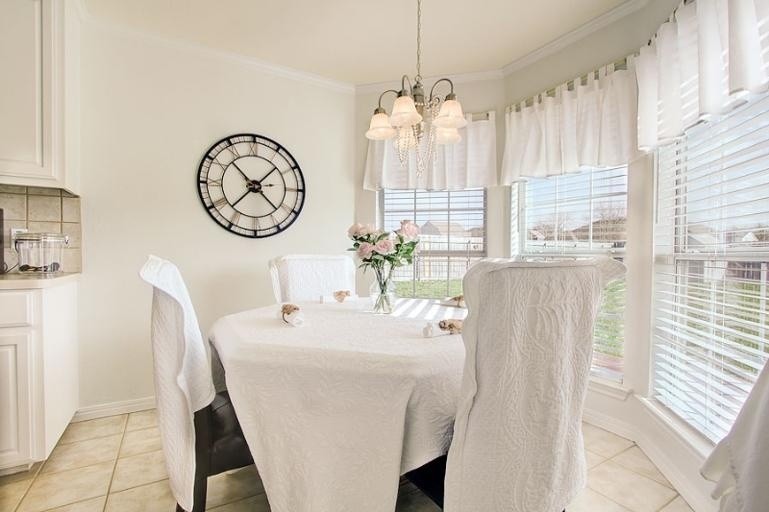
[0, 274, 84, 477]
[0, 1, 78, 196]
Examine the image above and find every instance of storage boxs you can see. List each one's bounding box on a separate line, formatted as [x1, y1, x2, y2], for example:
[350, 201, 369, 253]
[16, 234, 67, 273]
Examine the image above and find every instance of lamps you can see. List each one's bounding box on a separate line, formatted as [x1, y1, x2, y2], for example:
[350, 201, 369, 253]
[366, 0, 469, 178]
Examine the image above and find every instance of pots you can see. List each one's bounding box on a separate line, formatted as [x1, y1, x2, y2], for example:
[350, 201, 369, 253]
[13, 233, 67, 276]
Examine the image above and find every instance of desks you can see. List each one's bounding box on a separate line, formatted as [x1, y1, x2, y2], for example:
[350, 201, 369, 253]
[209, 293, 476, 512]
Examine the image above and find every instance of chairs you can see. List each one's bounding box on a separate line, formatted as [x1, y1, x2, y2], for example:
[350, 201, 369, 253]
[404, 252, 602, 509]
[141, 255, 256, 510]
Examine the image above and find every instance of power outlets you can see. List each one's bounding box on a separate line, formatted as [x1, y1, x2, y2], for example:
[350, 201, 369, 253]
[11, 229, 28, 249]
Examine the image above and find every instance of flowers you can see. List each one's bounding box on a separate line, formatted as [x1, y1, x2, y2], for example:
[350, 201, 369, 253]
[347, 220, 422, 313]
